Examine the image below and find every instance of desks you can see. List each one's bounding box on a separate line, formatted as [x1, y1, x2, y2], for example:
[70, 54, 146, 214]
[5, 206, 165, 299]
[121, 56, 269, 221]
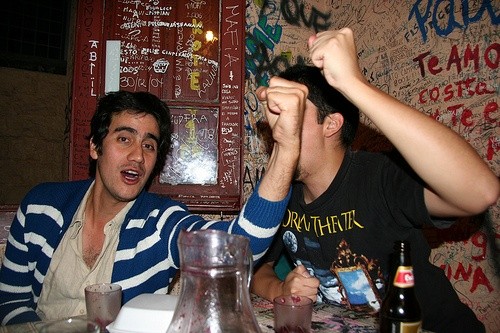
[0, 294, 380, 333]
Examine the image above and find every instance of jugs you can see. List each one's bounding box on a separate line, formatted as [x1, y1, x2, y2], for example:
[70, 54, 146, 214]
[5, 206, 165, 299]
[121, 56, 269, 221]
[165, 229, 263, 333]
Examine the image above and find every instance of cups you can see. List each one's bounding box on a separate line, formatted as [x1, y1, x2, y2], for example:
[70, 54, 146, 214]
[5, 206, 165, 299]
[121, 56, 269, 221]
[273, 295, 313, 333]
[84, 283, 122, 323]
[39, 318, 100, 333]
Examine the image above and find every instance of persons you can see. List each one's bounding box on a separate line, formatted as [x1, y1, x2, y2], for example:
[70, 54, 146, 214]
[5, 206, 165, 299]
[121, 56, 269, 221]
[0, 76, 309, 328]
[248, 26, 500, 333]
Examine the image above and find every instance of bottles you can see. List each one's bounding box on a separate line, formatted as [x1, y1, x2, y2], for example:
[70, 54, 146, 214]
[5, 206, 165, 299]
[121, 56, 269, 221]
[378, 241, 422, 333]
